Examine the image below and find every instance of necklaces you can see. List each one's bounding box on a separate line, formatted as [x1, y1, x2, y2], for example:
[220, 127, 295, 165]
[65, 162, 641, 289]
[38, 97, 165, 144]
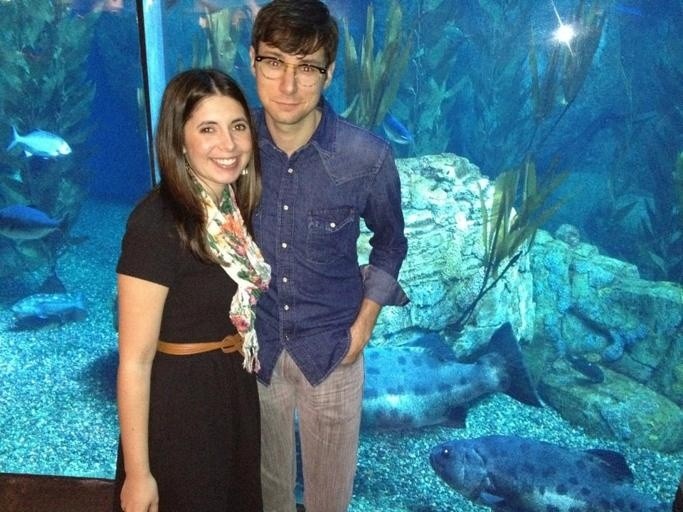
[192, 167, 219, 209]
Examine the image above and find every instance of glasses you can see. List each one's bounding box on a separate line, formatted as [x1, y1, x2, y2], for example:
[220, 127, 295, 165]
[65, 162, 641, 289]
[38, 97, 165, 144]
[255, 50, 329, 86]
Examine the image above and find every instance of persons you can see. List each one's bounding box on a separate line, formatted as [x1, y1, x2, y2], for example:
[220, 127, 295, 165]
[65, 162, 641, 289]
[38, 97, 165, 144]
[113, 67, 272, 511]
[244, 0, 410, 511]
